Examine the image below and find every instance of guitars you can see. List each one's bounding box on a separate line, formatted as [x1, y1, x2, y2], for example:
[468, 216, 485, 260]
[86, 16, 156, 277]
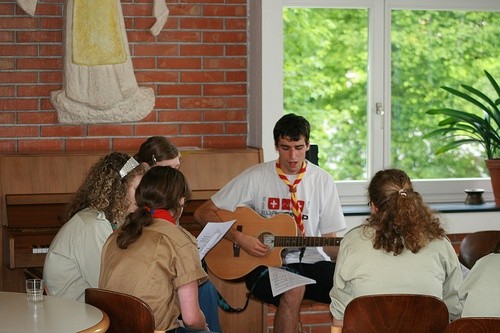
[202, 206, 344, 282]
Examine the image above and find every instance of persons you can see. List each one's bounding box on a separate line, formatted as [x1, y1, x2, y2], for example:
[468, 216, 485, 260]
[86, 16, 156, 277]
[457, 252, 500, 318]
[43, 137, 225, 333]
[193, 114, 343, 333]
[330, 168, 463, 333]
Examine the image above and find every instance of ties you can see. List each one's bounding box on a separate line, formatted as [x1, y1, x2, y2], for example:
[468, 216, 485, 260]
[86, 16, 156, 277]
[274, 159, 308, 237]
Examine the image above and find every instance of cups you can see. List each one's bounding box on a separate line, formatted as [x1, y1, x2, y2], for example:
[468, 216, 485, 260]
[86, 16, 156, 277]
[26, 279, 45, 304]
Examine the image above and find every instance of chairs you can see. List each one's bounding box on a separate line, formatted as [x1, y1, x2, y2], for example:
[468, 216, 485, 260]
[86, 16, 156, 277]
[342, 294, 449, 333]
[459, 230, 500, 270]
[85, 289, 154, 333]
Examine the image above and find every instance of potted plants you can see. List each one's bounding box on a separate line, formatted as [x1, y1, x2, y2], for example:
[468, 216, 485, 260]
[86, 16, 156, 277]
[421, 70, 500, 207]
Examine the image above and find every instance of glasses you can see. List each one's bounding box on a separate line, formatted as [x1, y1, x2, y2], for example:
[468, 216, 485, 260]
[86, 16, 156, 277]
[367, 201, 373, 207]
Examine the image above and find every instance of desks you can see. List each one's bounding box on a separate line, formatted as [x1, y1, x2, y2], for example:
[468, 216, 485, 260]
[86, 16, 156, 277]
[0, 291, 111, 333]
